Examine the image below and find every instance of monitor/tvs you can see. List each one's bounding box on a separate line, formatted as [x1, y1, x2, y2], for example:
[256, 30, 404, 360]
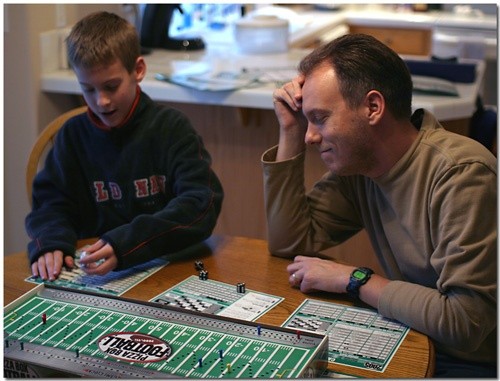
[169, 4, 242, 40]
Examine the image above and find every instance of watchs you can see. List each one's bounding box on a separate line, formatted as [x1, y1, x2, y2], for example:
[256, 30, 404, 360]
[346, 266, 376, 303]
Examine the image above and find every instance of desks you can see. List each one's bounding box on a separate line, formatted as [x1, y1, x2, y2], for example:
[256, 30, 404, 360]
[4, 238, 437, 379]
[40, 6, 497, 275]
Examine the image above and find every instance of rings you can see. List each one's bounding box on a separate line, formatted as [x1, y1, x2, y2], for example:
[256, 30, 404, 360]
[292, 273, 302, 282]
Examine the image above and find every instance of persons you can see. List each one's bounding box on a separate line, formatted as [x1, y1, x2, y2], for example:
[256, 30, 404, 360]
[261, 34, 498, 379]
[24, 11, 224, 281]
